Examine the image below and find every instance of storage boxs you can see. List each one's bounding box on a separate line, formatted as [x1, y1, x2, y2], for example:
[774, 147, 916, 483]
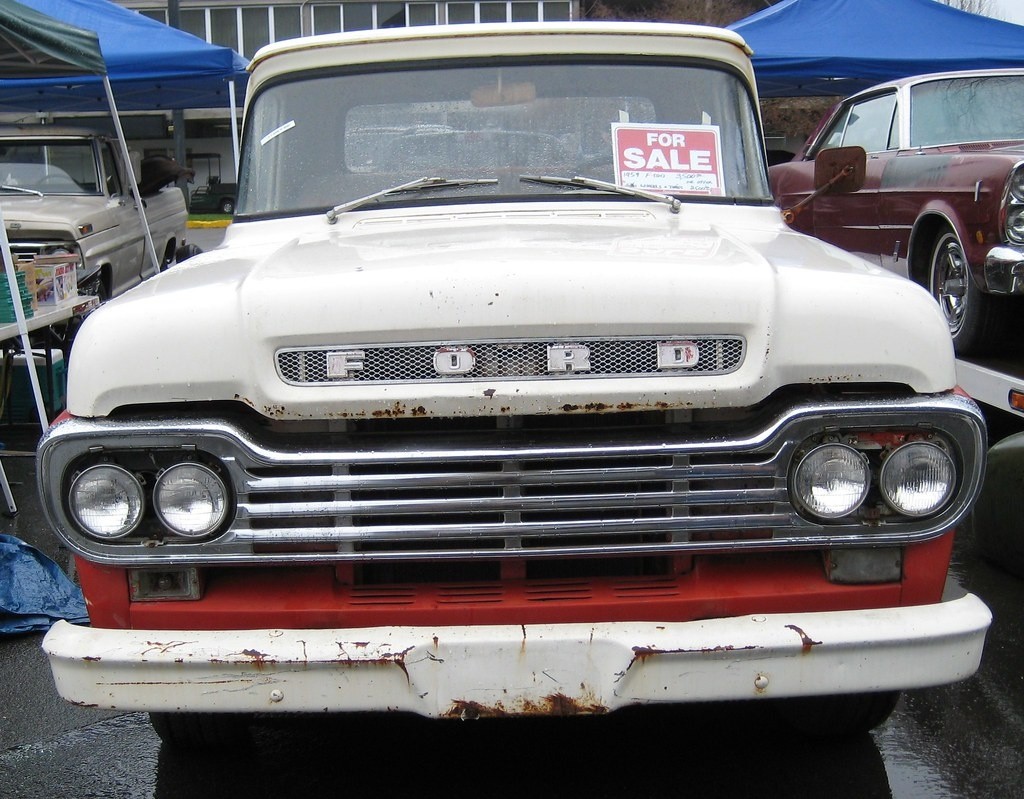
[34, 261, 77, 307]
[0, 349, 65, 424]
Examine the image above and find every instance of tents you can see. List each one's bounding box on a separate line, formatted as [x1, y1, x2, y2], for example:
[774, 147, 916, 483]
[0, 1, 254, 199]
[0, 0, 166, 516]
[717, 0, 1024, 99]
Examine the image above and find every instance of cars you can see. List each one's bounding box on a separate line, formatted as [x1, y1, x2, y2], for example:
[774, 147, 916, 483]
[34, 19, 994, 745]
[768, 67, 1023, 356]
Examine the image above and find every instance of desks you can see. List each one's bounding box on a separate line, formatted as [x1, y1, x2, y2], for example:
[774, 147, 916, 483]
[0, 295, 100, 457]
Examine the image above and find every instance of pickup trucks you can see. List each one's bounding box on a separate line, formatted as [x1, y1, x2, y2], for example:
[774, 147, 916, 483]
[1, 127, 190, 299]
